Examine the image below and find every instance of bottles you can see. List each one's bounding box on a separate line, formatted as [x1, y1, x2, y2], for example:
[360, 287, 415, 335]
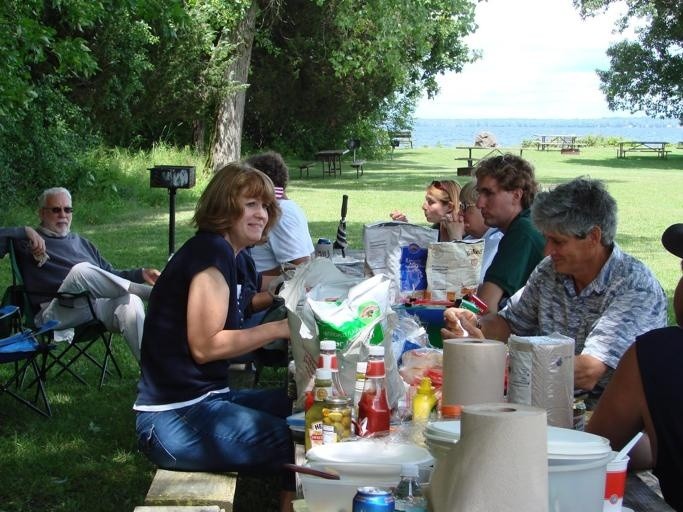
[304, 339, 464, 453]
[391, 464, 427, 511]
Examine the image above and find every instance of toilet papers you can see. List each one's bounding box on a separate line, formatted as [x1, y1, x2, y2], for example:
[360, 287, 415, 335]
[442, 340, 507, 406]
[432, 405, 551, 507]
[506, 333, 573, 429]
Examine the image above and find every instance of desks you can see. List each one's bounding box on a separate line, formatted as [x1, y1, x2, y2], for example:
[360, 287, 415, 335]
[314, 148, 350, 179]
[619, 141, 669, 158]
[455, 146, 532, 176]
[533, 133, 576, 149]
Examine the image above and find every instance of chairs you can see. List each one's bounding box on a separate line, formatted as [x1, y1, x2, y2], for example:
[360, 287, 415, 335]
[8, 237, 122, 389]
[1, 304, 52, 418]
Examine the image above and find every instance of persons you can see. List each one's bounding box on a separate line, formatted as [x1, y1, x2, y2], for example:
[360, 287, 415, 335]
[230, 150, 315, 365]
[585, 222, 683, 511]
[441, 179, 504, 298]
[469, 153, 550, 316]
[389, 179, 463, 242]
[0, 187, 162, 368]
[132, 161, 297, 511]
[439, 174, 669, 395]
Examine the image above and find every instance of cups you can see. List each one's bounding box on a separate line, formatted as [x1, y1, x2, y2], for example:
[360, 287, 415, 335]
[605, 450, 631, 512]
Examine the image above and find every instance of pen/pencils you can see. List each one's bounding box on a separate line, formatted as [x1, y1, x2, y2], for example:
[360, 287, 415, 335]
[285, 463, 340, 482]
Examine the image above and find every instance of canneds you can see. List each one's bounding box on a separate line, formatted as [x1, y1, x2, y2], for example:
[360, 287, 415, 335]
[351, 485, 395, 512]
[322, 394, 353, 443]
[572, 400, 586, 433]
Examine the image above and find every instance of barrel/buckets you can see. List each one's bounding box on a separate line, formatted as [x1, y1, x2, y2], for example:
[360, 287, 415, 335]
[423, 421, 611, 510]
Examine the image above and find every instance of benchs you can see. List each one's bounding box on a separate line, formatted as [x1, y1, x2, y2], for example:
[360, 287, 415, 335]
[388, 130, 412, 148]
[300, 163, 315, 178]
[135, 504, 224, 512]
[351, 160, 365, 178]
[623, 150, 672, 153]
[455, 158, 480, 162]
[146, 467, 238, 512]
[538, 142, 586, 149]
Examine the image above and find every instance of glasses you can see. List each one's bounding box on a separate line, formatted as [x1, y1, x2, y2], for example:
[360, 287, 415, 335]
[42, 207, 74, 213]
[460, 202, 477, 212]
[432, 181, 451, 201]
